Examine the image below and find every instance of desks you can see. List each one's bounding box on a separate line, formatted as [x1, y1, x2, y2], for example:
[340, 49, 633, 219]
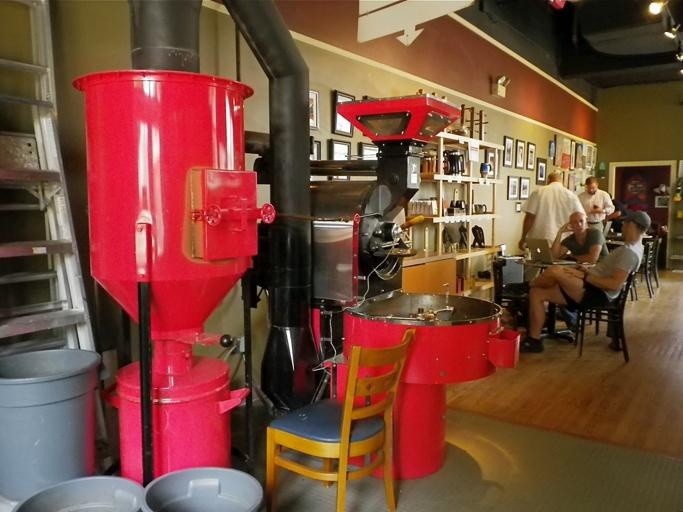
[607, 232, 651, 247]
[516, 259, 584, 347]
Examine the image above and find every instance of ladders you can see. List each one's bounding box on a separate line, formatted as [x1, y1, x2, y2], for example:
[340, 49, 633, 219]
[0, 0, 115, 477]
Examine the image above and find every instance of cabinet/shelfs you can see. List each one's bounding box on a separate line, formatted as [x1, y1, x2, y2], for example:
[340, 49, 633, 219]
[401, 91, 504, 304]
[663, 186, 682, 274]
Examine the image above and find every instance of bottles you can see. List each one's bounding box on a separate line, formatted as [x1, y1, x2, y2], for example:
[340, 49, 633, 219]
[420, 157, 436, 175]
[522, 246, 530, 260]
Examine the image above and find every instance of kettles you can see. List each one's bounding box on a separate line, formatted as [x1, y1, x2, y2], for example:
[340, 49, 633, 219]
[445, 150, 465, 174]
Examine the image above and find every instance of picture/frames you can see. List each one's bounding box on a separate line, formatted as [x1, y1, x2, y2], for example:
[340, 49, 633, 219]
[654, 193, 670, 209]
[306, 86, 380, 184]
[501, 130, 599, 214]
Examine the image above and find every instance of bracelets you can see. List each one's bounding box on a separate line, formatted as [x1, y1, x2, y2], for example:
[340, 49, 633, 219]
[583, 270, 588, 281]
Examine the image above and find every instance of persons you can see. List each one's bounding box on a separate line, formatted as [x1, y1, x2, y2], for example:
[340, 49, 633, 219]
[503, 211, 651, 353]
[552, 212, 609, 339]
[603, 193, 628, 232]
[518, 171, 587, 255]
[578, 177, 615, 256]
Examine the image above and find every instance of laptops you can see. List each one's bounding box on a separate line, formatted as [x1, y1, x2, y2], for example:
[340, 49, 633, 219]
[526, 237, 577, 266]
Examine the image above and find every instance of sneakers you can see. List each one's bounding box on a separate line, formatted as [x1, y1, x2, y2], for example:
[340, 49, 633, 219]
[504, 281, 530, 296]
[519, 335, 544, 353]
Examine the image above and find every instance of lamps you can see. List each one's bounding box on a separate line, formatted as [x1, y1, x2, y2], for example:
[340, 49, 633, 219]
[646, 0, 682, 80]
[490, 74, 511, 102]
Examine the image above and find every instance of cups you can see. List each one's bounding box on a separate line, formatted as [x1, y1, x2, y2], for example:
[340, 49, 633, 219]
[443, 240, 460, 254]
[479, 163, 492, 173]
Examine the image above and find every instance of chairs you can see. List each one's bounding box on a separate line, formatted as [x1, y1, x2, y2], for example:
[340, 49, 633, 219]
[571, 269, 639, 363]
[260, 329, 418, 512]
[629, 239, 655, 302]
[632, 233, 665, 288]
[485, 242, 535, 343]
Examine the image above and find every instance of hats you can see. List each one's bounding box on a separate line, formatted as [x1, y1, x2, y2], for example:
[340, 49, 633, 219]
[625, 209, 651, 227]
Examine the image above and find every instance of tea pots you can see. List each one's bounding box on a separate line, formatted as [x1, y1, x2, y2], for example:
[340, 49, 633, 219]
[472, 204, 486, 214]
